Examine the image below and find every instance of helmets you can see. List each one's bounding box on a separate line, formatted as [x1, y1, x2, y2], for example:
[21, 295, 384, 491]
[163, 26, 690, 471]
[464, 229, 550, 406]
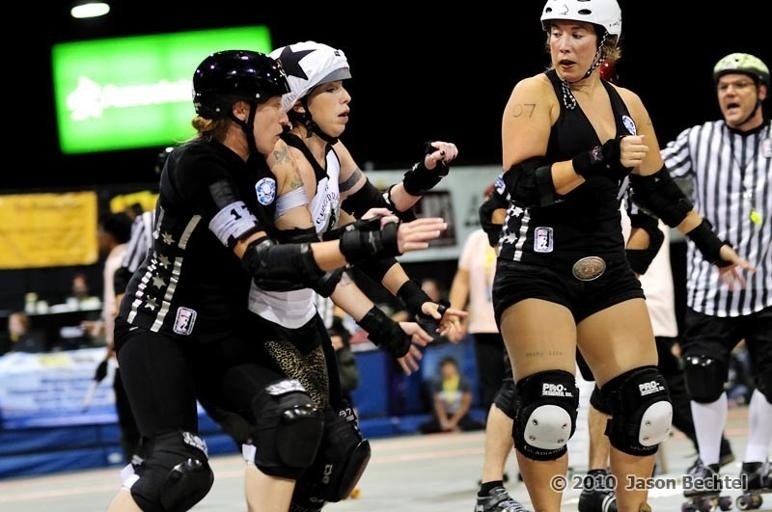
[193, 50, 291, 120]
[712, 53, 770, 82]
[539, 0, 623, 48]
[267, 41, 352, 114]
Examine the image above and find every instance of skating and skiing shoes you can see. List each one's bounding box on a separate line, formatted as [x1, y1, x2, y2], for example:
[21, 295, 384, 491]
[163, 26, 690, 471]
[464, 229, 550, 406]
[735, 461, 772, 511]
[681, 458, 732, 512]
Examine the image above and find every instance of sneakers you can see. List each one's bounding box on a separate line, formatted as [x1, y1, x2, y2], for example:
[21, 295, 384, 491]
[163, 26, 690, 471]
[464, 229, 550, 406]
[578, 481, 616, 512]
[473, 487, 529, 512]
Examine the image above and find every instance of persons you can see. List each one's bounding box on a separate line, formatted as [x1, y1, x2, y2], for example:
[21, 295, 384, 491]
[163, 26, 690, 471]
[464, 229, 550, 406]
[1, 0, 771, 511]
